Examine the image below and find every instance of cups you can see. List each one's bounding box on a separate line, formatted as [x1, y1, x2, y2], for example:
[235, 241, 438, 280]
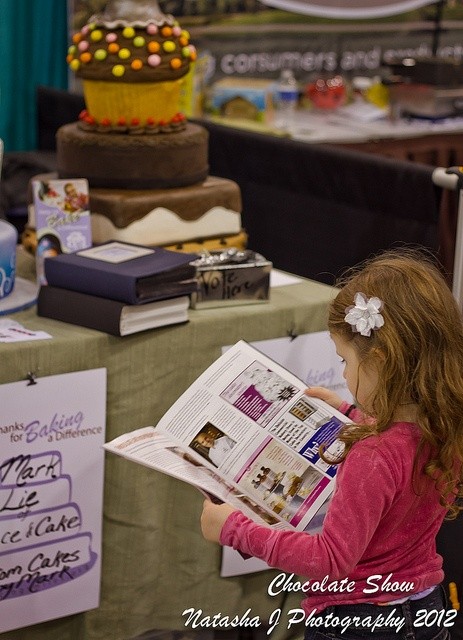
[0, 217, 18, 299]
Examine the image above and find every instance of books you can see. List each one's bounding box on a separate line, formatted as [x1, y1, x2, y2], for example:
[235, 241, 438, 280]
[44, 240, 201, 304]
[36, 284, 191, 337]
[103, 339, 358, 560]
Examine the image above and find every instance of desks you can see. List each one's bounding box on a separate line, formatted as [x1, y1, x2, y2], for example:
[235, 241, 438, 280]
[260, 101, 462, 291]
[0, 248, 346, 637]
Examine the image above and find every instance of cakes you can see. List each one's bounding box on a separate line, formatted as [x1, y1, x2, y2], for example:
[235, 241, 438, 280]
[22, 13, 249, 252]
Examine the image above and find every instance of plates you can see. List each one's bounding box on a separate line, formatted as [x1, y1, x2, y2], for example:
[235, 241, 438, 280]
[0, 274, 38, 313]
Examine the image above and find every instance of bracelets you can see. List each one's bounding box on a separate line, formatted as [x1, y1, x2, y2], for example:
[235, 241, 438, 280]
[345, 402, 356, 417]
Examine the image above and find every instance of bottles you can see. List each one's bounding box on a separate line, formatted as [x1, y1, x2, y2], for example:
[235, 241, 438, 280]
[276, 70, 302, 133]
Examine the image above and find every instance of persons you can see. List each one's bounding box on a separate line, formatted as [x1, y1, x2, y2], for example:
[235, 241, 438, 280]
[200, 245, 462, 639]
